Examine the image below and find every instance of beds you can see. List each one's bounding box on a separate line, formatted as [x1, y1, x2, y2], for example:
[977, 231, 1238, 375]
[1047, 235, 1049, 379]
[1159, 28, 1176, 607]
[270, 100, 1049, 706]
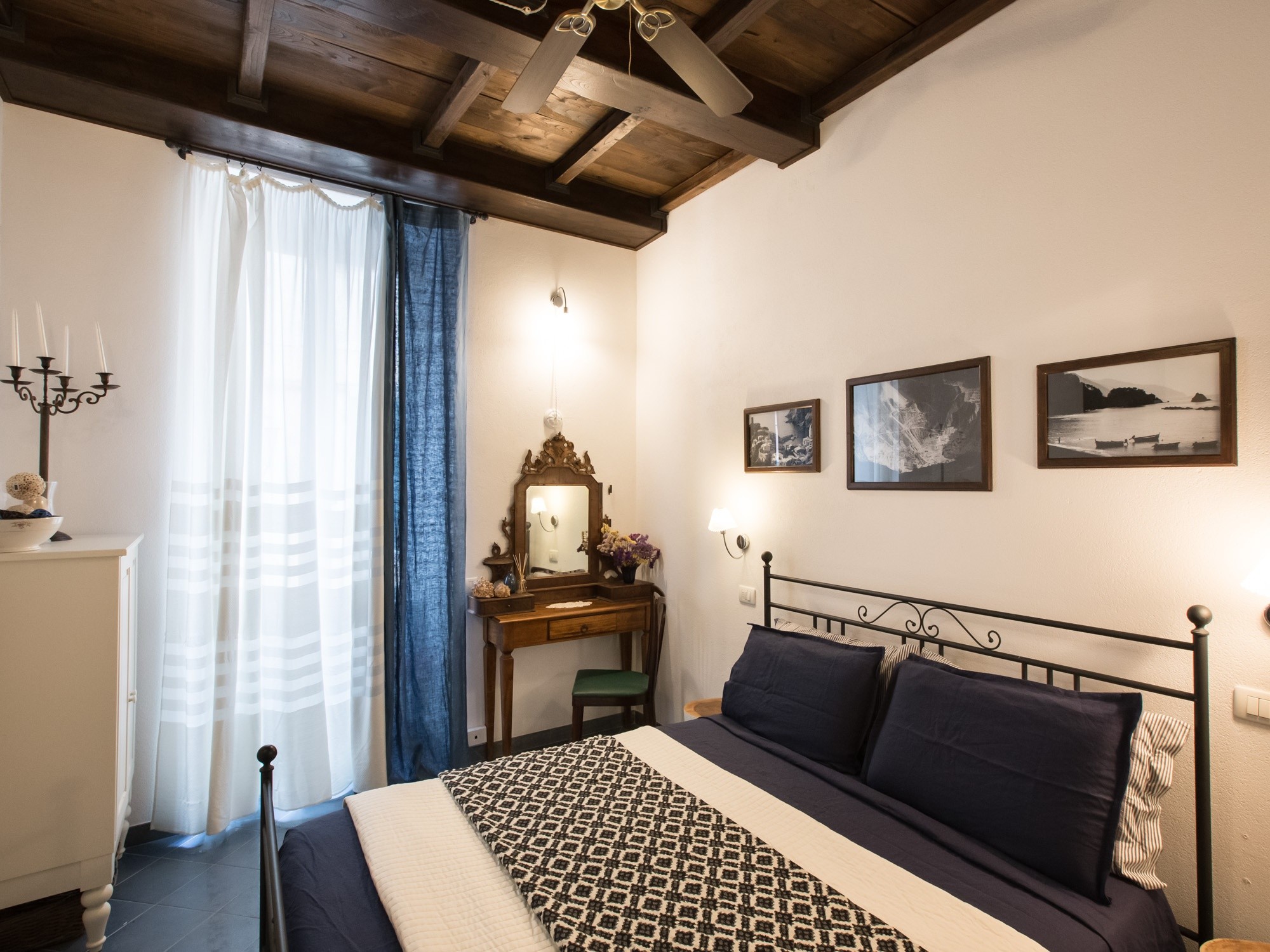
[257, 552, 1214, 952]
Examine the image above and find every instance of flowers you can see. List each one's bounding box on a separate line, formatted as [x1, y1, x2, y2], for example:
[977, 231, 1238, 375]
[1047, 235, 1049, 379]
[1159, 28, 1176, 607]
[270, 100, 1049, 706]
[596, 521, 660, 569]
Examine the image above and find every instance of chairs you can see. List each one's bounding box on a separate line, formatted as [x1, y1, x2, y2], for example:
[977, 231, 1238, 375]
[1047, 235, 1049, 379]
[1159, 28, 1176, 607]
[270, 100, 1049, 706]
[570, 597, 667, 741]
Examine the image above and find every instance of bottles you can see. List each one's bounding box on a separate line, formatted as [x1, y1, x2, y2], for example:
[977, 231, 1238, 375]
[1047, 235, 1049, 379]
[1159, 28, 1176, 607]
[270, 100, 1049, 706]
[505, 570, 518, 594]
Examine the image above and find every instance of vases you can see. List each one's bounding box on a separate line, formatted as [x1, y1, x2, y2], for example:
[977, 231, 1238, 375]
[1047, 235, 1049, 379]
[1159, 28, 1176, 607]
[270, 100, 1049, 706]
[620, 566, 638, 583]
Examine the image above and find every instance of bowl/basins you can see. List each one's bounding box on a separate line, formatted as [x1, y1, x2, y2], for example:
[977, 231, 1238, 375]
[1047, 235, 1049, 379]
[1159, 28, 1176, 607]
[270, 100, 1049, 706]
[0, 516, 64, 555]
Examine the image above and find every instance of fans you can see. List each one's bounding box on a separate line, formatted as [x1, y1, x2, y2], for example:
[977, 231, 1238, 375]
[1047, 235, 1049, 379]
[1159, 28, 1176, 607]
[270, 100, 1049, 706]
[486, 0, 754, 119]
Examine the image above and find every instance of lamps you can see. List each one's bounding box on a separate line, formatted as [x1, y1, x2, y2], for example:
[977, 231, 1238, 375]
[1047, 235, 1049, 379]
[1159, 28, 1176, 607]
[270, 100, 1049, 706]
[708, 507, 749, 559]
[531, 497, 558, 532]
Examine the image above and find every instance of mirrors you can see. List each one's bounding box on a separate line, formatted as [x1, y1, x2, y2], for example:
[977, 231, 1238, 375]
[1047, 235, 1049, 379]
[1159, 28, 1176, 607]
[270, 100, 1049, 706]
[524, 483, 591, 579]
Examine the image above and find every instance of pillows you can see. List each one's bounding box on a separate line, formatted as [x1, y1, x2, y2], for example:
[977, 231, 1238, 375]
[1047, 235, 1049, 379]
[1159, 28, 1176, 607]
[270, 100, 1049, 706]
[720, 623, 886, 773]
[860, 653, 1143, 903]
[917, 649, 1192, 890]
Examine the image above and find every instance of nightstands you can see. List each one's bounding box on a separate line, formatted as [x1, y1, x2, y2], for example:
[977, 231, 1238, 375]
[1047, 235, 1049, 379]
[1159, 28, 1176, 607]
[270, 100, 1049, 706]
[684, 698, 723, 721]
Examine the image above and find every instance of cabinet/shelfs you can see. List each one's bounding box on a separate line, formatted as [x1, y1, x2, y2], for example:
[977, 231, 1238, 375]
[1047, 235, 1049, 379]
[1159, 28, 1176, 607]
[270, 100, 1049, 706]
[0, 532, 145, 952]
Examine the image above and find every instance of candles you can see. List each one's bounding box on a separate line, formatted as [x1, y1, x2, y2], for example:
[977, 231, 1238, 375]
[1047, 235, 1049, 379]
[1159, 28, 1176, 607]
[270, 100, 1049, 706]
[8, 305, 109, 378]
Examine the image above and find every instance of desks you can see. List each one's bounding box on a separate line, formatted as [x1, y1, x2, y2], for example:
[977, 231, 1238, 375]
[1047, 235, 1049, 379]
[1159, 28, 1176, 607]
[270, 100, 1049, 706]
[467, 579, 656, 759]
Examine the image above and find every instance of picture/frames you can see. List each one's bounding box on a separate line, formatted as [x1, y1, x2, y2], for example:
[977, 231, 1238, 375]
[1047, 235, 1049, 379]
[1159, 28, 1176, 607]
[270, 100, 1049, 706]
[846, 355, 993, 492]
[771, 618, 919, 703]
[1036, 335, 1238, 470]
[743, 398, 821, 472]
